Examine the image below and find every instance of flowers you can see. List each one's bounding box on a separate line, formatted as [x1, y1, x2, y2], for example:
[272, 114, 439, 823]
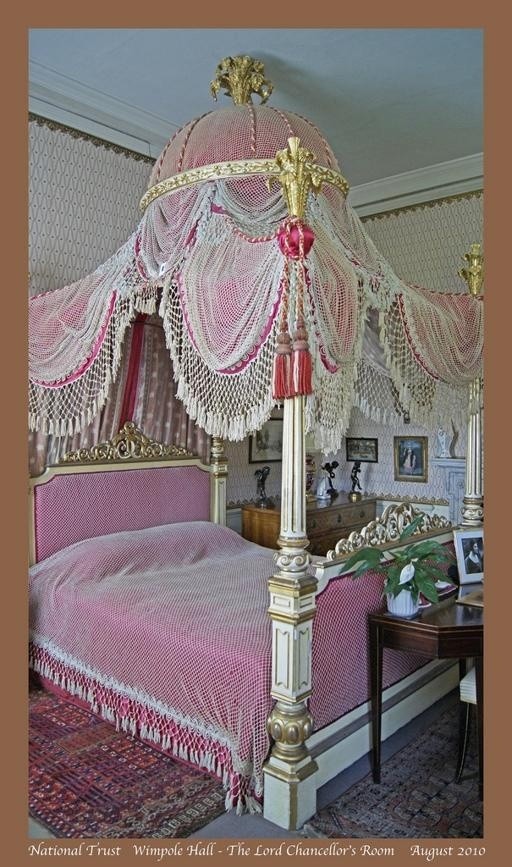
[337, 515, 454, 604]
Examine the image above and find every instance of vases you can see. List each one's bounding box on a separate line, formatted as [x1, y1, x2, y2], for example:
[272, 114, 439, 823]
[383, 579, 420, 618]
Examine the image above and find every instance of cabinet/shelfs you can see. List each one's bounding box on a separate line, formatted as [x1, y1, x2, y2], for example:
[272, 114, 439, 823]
[242, 494, 377, 554]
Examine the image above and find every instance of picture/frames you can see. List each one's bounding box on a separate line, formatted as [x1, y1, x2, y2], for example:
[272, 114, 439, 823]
[346, 437, 378, 463]
[453, 529, 484, 584]
[249, 417, 283, 463]
[393, 436, 429, 483]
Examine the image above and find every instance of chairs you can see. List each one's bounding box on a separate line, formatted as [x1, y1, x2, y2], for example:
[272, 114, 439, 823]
[453, 664, 478, 783]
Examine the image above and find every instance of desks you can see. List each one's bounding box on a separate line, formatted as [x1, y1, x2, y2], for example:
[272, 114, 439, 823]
[366, 572, 483, 783]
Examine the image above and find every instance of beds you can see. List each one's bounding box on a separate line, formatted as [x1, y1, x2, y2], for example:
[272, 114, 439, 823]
[29, 50, 483, 832]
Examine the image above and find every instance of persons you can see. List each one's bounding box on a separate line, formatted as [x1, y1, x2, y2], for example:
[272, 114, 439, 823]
[463, 539, 483, 573]
[348, 461, 361, 493]
[402, 443, 415, 474]
[254, 466, 271, 497]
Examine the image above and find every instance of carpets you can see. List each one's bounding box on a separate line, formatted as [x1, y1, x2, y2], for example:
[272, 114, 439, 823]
[29, 677, 230, 838]
[302, 697, 484, 839]
